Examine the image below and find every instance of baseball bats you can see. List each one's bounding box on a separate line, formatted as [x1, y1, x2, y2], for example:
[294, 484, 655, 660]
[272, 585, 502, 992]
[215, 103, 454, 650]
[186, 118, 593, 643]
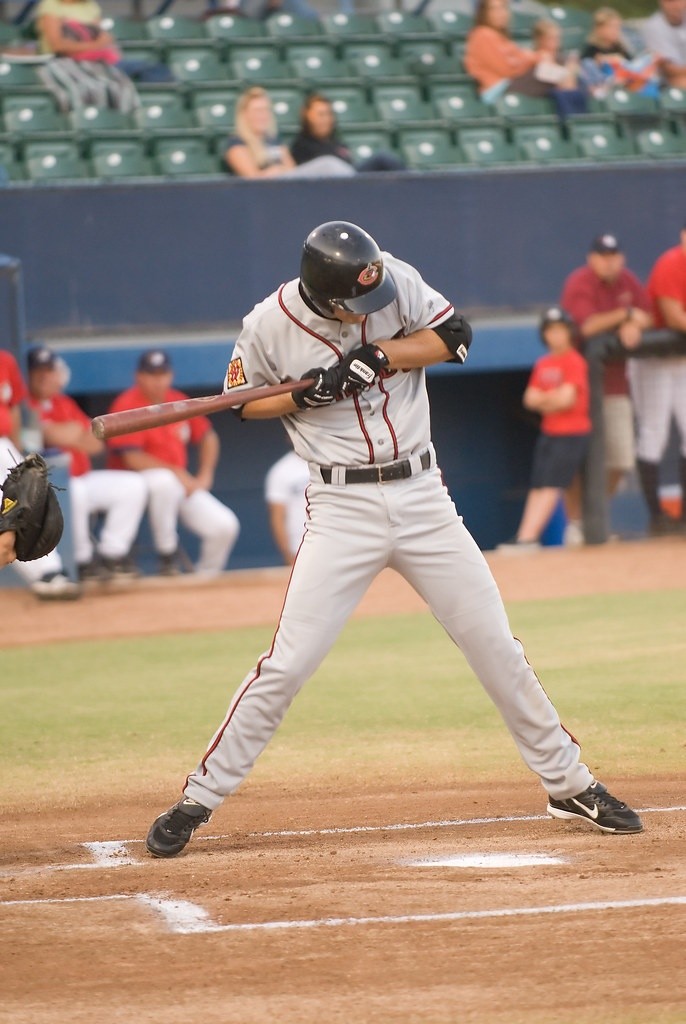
[90, 378, 316, 439]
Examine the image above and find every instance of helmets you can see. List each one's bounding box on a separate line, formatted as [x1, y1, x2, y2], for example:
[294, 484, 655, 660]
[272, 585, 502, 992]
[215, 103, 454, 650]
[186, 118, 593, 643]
[300, 220, 397, 315]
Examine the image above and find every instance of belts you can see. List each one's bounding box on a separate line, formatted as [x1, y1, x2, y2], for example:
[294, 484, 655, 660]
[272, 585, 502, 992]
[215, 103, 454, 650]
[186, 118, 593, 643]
[321, 452, 430, 484]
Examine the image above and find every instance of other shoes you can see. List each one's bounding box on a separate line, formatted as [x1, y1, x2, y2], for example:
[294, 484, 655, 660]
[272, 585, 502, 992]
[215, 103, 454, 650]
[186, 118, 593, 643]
[32, 573, 80, 598]
[77, 560, 104, 581]
[162, 546, 195, 576]
[96, 551, 144, 576]
[497, 537, 541, 550]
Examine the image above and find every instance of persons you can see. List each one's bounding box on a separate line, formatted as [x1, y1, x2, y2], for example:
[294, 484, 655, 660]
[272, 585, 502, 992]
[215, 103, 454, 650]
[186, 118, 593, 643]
[559, 234, 655, 544]
[0, 351, 78, 600]
[222, 86, 357, 176]
[463, 0, 686, 100]
[624, 224, 686, 519]
[0, 457, 63, 569]
[265, 448, 311, 564]
[28, 347, 147, 585]
[105, 349, 240, 576]
[496, 305, 589, 549]
[147, 221, 645, 857]
[35, 0, 141, 116]
[292, 95, 402, 170]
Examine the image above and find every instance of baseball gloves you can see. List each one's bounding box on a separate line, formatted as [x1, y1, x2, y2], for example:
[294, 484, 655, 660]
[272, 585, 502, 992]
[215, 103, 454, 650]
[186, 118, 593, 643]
[0, 451, 64, 562]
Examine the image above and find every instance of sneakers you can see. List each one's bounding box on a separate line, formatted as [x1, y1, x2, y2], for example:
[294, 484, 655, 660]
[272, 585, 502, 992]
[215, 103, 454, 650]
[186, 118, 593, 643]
[146, 795, 213, 857]
[547, 777, 642, 833]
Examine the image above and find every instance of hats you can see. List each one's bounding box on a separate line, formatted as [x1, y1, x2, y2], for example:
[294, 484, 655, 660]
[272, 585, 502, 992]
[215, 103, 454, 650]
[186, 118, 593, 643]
[590, 229, 629, 255]
[27, 347, 56, 368]
[137, 351, 169, 372]
[539, 308, 571, 330]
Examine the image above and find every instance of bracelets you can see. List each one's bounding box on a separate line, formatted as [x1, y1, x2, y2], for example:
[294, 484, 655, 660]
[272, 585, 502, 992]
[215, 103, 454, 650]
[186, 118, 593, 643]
[628, 306, 634, 320]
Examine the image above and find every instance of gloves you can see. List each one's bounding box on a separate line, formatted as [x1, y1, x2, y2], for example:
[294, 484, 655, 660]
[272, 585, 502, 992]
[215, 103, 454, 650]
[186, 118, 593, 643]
[333, 343, 388, 401]
[291, 366, 339, 411]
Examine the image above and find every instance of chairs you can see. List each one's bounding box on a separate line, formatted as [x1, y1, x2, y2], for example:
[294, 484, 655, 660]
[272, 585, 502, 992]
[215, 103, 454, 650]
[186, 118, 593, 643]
[0, 0, 686, 182]
